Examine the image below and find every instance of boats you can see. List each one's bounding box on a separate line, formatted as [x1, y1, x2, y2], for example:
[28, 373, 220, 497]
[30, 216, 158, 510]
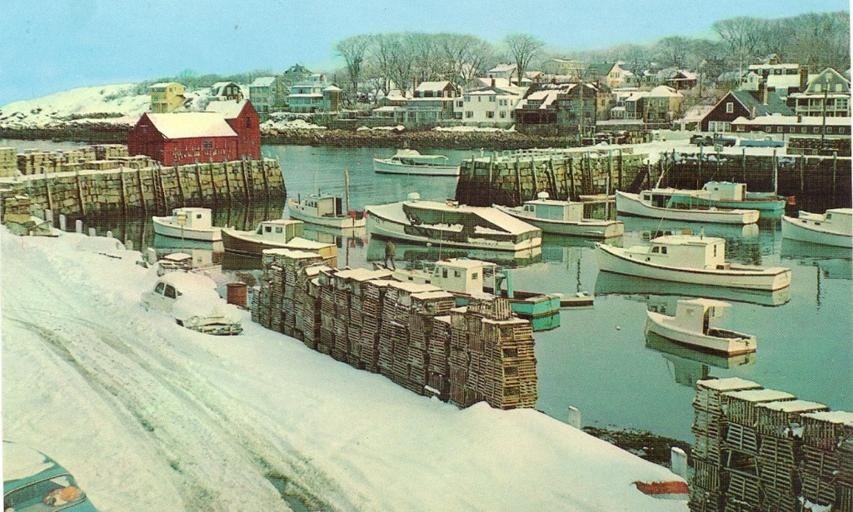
[373, 149, 460, 174]
[152, 170, 365, 260]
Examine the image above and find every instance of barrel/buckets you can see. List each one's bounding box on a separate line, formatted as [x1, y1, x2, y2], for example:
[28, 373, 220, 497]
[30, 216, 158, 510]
[227, 282, 247, 306]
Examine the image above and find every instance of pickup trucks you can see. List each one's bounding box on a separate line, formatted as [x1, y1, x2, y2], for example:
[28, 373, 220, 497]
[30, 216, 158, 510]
[740, 135, 784, 147]
[697, 132, 736, 147]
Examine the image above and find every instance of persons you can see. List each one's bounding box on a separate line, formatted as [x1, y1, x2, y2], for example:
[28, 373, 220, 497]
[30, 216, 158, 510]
[383, 239, 397, 270]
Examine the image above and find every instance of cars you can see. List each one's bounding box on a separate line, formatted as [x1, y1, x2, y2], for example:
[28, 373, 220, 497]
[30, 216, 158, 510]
[690, 134, 703, 144]
[3, 440, 102, 512]
[141, 272, 243, 336]
